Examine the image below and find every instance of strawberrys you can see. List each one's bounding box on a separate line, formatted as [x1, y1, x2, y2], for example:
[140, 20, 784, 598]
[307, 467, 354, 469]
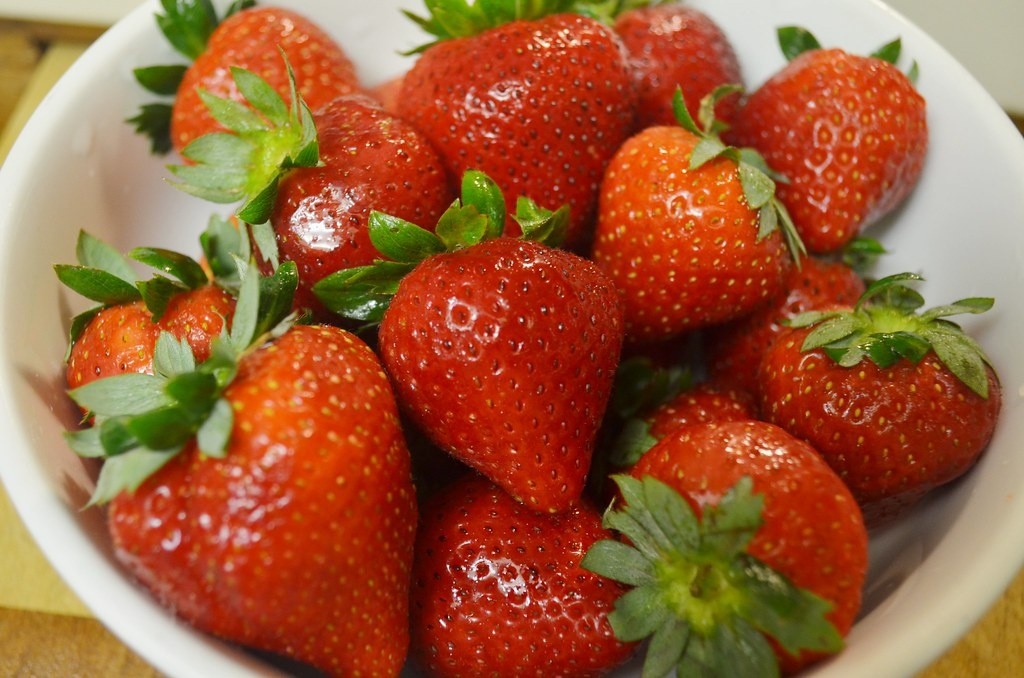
[62, 2, 1000, 677]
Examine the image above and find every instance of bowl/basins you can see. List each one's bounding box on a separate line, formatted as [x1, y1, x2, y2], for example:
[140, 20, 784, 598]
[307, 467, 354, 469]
[0, 0, 1024, 678]
